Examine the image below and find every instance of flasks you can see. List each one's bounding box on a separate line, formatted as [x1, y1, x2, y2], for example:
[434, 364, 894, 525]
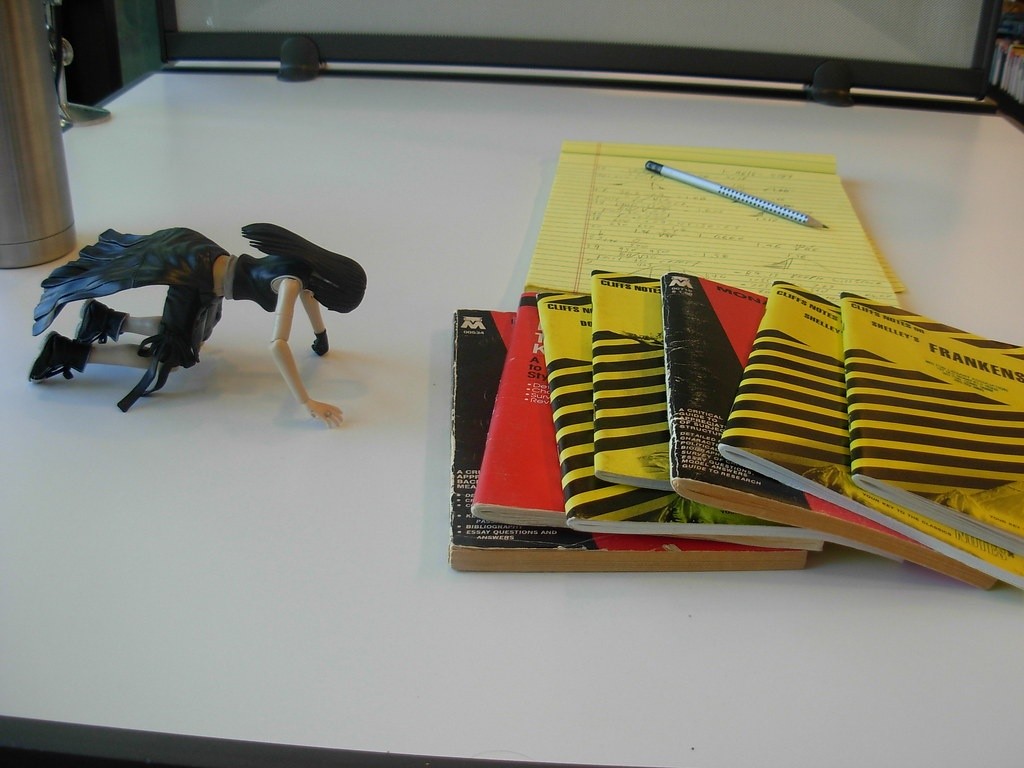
[0, 0, 75, 269]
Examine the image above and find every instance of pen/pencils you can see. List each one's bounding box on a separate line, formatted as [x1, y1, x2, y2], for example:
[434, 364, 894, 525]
[644, 160, 830, 230]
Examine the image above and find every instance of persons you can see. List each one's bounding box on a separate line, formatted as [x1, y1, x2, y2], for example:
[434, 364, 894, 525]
[29, 223, 367, 430]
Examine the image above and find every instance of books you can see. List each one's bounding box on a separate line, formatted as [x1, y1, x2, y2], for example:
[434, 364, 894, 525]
[449, 36, 1024, 592]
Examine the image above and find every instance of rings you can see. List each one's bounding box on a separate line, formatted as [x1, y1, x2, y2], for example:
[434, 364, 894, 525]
[324, 411, 332, 417]
